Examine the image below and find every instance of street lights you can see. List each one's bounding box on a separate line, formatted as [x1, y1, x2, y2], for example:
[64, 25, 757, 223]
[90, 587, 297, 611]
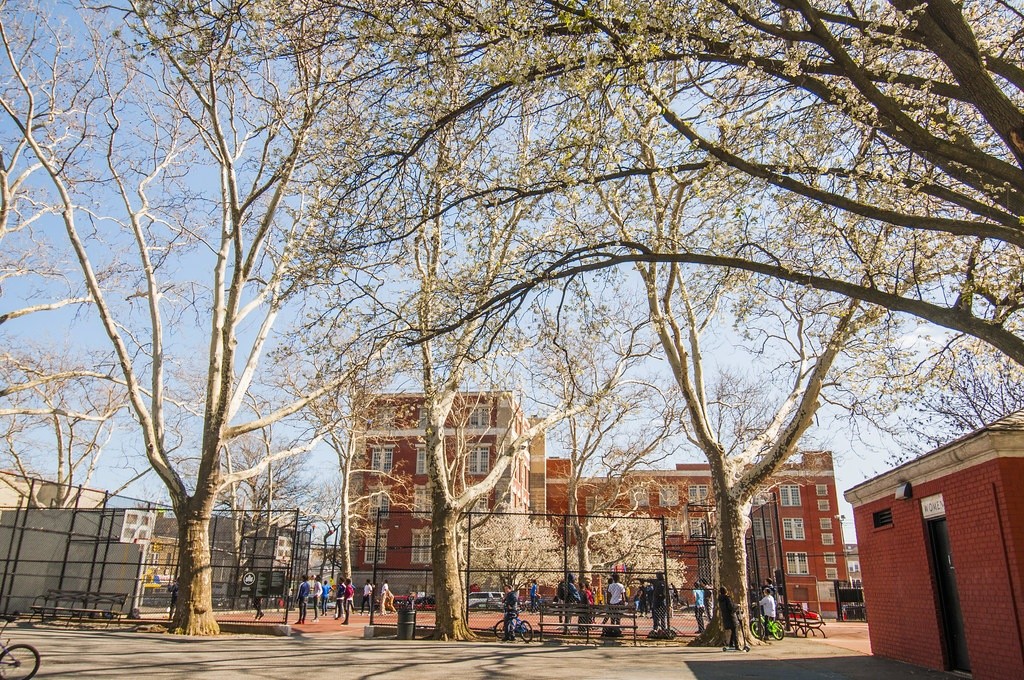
[834, 514, 853, 588]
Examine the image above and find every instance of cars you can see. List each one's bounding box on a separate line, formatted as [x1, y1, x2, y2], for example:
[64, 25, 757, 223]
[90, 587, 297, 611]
[374, 595, 437, 611]
[789, 608, 820, 620]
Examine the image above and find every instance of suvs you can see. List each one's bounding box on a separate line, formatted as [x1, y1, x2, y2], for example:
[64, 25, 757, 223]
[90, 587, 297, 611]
[842, 605, 866, 621]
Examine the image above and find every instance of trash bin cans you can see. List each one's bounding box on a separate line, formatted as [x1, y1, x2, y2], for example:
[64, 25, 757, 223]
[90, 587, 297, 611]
[397, 611, 416, 640]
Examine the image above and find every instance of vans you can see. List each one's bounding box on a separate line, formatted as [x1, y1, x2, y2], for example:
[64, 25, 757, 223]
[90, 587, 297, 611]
[468, 591, 505, 609]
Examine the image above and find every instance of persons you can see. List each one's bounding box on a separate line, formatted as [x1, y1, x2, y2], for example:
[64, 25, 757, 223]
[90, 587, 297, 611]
[379, 579, 388, 616]
[253, 597, 263, 620]
[558, 574, 597, 630]
[718, 586, 742, 651]
[502, 584, 517, 641]
[751, 578, 776, 640]
[633, 571, 678, 630]
[167, 577, 180, 620]
[602, 574, 626, 624]
[295, 575, 310, 624]
[529, 582, 541, 614]
[386, 590, 396, 614]
[341, 579, 354, 625]
[335, 577, 346, 620]
[311, 575, 323, 622]
[320, 580, 333, 616]
[358, 579, 373, 616]
[692, 578, 712, 633]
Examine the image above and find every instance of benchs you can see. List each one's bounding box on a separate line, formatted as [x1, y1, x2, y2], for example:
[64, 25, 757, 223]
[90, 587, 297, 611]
[28, 588, 130, 628]
[537, 603, 638, 644]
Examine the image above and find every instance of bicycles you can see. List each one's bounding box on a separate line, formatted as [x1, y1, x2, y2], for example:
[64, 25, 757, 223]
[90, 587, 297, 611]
[751, 603, 785, 641]
[0, 615, 40, 680]
[494, 605, 534, 643]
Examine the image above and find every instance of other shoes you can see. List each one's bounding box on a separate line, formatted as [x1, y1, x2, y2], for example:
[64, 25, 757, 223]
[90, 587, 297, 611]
[295, 619, 305, 624]
[311, 618, 319, 623]
[695, 630, 703, 634]
[341, 620, 349, 625]
[735, 646, 743, 651]
[557, 626, 563, 630]
[729, 643, 736, 647]
[763, 636, 770, 640]
[321, 610, 396, 619]
[502, 636, 515, 641]
[255, 613, 264, 619]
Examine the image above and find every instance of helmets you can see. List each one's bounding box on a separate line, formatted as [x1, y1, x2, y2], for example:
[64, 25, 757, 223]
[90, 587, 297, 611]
[508, 610, 519, 619]
